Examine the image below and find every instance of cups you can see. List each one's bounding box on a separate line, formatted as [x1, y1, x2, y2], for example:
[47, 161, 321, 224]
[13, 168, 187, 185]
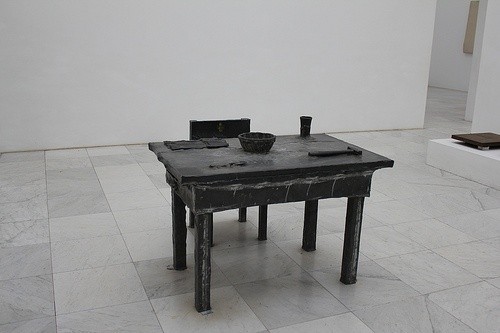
[299, 115, 312, 137]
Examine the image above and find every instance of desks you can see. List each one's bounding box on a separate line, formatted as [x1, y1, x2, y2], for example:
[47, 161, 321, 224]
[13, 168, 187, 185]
[148, 132, 395, 316]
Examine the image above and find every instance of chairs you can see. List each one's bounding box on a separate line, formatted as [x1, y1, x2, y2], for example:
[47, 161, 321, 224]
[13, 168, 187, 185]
[186, 117, 270, 247]
